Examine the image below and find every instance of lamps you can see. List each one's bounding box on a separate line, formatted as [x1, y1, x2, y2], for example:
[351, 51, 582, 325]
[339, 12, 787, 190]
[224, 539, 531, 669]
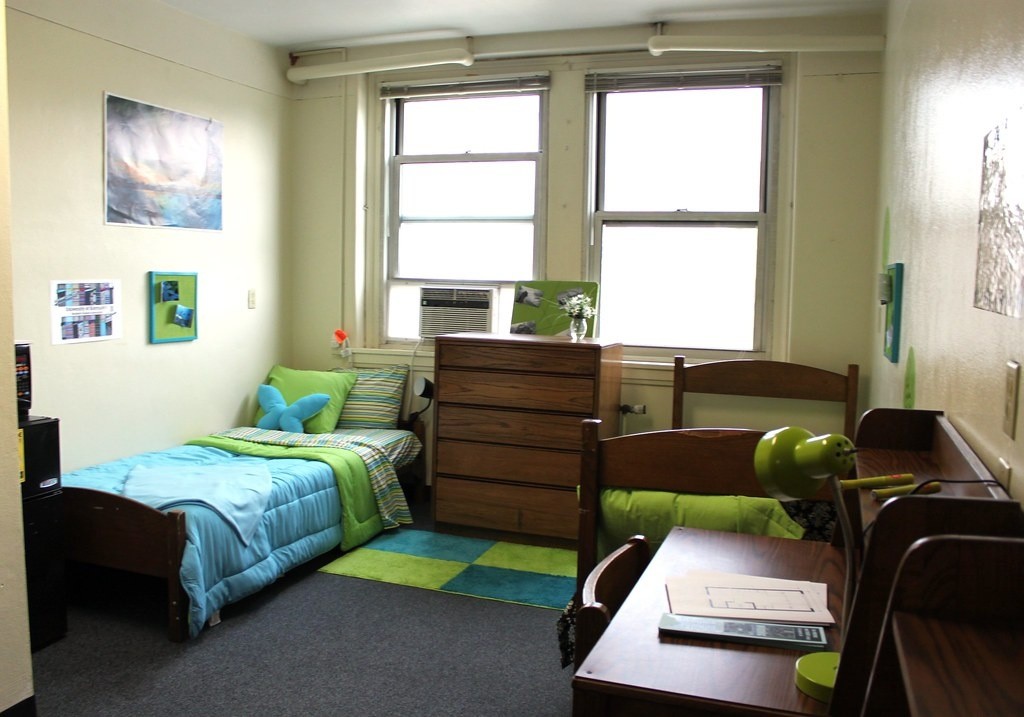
[407, 377, 434, 426]
[754, 427, 861, 704]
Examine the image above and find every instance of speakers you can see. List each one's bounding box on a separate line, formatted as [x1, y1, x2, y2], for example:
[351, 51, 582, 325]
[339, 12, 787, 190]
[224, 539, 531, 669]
[19, 416, 62, 502]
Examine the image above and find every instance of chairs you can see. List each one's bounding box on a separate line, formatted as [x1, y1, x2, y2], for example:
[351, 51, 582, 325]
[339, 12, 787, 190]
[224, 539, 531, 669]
[573, 536, 652, 670]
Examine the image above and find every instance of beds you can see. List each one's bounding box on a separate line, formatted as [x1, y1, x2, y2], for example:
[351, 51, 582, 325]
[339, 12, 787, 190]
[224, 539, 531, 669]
[59, 419, 426, 641]
[579, 356, 872, 593]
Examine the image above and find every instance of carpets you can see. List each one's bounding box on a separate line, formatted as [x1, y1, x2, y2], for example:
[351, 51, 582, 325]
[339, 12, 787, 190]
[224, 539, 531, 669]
[314, 523, 582, 611]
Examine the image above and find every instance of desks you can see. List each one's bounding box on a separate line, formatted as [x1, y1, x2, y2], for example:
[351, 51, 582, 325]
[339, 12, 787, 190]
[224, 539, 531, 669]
[574, 523, 846, 717]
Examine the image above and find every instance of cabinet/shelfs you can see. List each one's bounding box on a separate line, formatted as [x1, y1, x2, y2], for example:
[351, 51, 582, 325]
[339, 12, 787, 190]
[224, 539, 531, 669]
[832, 407, 1023, 717]
[433, 333, 622, 545]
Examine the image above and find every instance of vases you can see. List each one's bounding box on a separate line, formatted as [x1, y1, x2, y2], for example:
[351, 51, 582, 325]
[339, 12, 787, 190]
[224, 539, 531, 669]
[570, 319, 587, 340]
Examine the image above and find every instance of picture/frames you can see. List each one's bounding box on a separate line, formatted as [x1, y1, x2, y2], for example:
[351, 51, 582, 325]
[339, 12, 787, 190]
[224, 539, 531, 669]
[150, 270, 197, 343]
[882, 262, 904, 365]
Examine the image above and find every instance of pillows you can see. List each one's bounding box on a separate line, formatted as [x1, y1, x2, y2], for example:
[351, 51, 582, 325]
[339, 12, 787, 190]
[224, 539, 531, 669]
[325, 362, 409, 430]
[253, 364, 358, 435]
[570, 484, 806, 564]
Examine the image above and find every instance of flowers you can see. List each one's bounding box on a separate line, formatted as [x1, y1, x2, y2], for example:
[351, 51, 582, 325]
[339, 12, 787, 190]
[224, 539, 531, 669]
[563, 293, 597, 319]
